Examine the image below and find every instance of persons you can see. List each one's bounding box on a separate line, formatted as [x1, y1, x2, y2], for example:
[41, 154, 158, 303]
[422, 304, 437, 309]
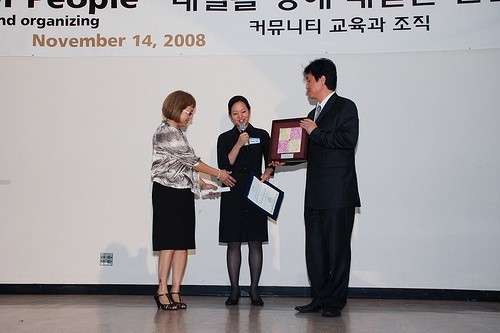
[266, 58, 361, 317]
[216, 96, 276, 307]
[151, 90, 236, 310]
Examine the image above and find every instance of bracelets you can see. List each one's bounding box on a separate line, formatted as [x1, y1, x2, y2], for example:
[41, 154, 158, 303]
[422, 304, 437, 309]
[201, 183, 206, 189]
[217, 169, 220, 178]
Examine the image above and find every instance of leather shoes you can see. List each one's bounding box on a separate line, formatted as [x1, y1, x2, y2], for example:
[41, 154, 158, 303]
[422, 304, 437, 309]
[295, 302, 323, 313]
[322, 306, 342, 317]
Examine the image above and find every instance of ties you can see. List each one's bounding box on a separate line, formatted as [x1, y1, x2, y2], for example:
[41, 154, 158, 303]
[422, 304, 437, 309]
[314, 105, 321, 122]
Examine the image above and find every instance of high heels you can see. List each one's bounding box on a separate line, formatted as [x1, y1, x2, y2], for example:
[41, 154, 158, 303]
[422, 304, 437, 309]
[169, 291, 187, 309]
[249, 289, 264, 306]
[154, 291, 177, 310]
[225, 290, 241, 306]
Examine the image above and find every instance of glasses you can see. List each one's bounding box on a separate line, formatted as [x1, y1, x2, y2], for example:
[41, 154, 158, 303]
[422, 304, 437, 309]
[183, 110, 194, 115]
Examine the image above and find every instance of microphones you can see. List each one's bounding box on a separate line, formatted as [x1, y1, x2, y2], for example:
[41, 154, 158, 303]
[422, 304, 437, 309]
[239, 123, 250, 152]
